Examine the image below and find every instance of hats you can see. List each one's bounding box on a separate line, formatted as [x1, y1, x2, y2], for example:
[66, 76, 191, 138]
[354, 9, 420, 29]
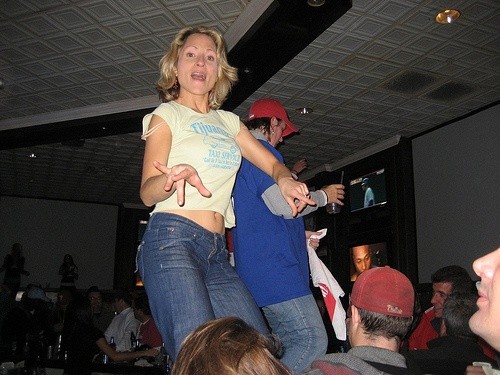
[249, 99, 300, 138]
[352, 266, 415, 318]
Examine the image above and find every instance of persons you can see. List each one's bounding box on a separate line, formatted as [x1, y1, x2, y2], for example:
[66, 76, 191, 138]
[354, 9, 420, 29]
[361, 180, 374, 208]
[350, 244, 378, 281]
[0, 239, 500, 375]
[134, 26, 344, 375]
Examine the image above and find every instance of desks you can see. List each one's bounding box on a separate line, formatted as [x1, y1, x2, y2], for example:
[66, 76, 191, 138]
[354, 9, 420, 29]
[0, 348, 167, 375]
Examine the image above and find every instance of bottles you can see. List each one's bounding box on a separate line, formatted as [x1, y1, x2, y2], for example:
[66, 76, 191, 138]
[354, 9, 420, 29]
[129, 332, 138, 352]
[108, 336, 116, 353]
[160, 343, 167, 370]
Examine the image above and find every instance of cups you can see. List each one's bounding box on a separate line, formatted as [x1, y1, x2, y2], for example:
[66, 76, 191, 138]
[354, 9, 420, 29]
[100, 353, 110, 364]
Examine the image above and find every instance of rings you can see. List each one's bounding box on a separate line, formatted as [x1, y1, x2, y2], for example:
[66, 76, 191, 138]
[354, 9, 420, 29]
[306, 192, 310, 196]
